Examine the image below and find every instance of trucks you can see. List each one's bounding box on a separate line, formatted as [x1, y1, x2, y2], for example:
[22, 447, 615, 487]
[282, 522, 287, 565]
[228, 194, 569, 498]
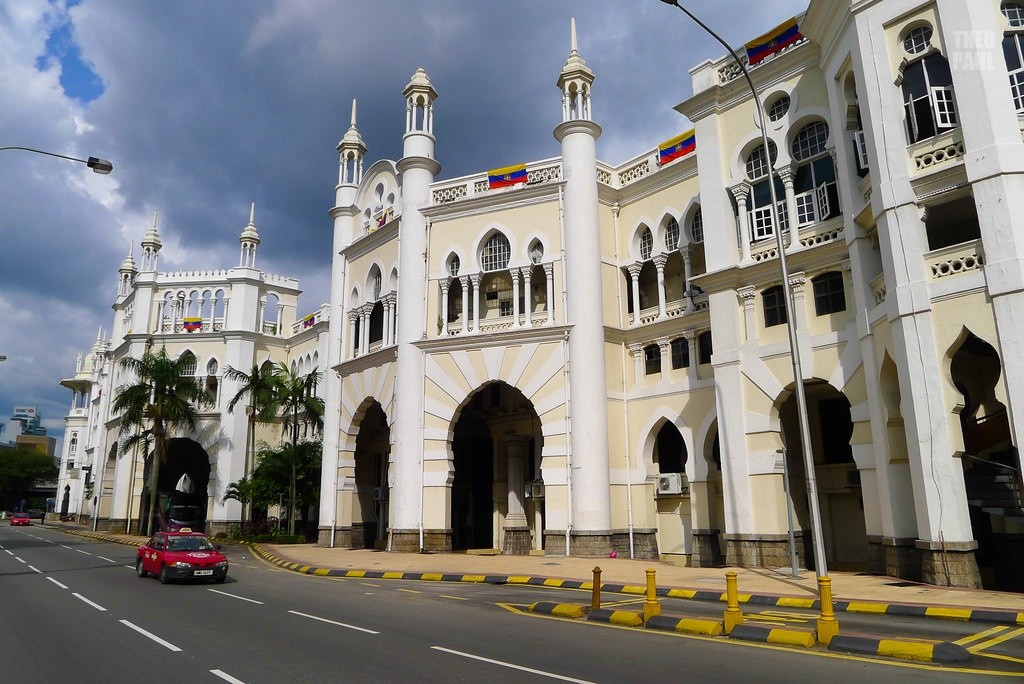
[144, 491, 203, 538]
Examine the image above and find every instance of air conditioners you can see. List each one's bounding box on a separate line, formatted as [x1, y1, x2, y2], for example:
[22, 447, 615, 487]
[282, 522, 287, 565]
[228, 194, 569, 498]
[658, 473, 682, 494]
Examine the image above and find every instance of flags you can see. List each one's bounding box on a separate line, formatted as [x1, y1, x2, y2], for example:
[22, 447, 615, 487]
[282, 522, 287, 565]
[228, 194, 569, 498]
[655, 127, 696, 164]
[742, 15, 802, 64]
[487, 163, 527, 189]
[303, 313, 314, 327]
[183, 317, 202, 328]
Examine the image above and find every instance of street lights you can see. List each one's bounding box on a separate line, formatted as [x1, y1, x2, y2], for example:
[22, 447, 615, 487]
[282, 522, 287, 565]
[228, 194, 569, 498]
[662, 0, 830, 593]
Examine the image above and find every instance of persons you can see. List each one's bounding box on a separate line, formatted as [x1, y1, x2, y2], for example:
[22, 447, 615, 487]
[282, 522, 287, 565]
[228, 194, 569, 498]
[40, 511, 45, 525]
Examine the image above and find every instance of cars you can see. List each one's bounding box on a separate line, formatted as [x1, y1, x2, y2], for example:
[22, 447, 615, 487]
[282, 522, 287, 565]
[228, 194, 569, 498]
[136, 528, 228, 584]
[9, 513, 29, 525]
